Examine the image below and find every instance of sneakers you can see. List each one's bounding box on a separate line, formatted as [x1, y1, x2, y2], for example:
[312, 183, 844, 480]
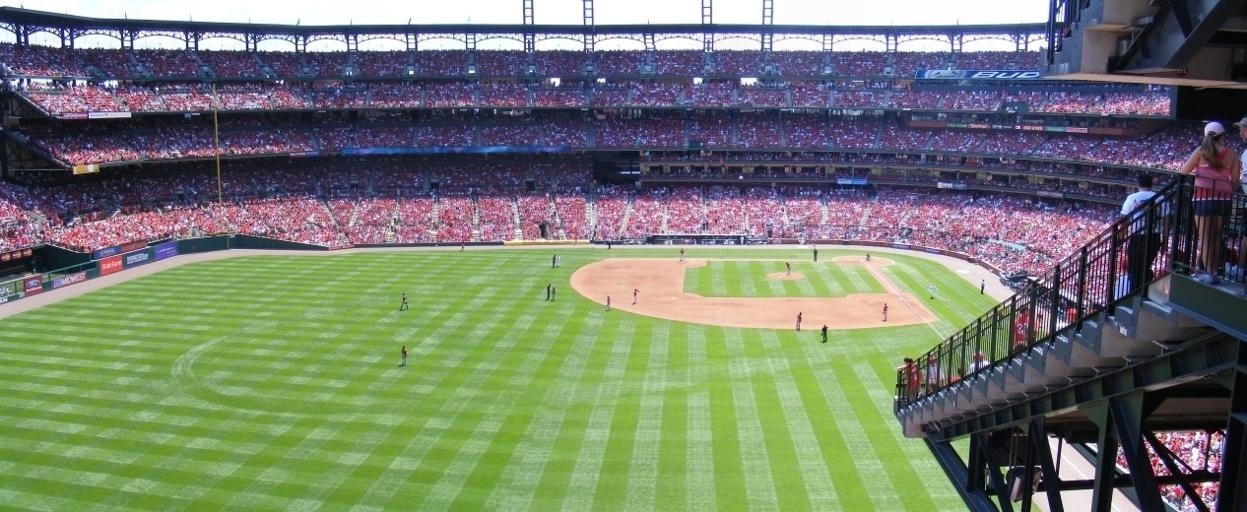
[1225, 265, 1243, 280]
[1192, 270, 1217, 284]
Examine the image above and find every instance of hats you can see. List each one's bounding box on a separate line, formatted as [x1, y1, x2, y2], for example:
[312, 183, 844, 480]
[1232, 118, 1247, 128]
[1204, 122, 1224, 137]
